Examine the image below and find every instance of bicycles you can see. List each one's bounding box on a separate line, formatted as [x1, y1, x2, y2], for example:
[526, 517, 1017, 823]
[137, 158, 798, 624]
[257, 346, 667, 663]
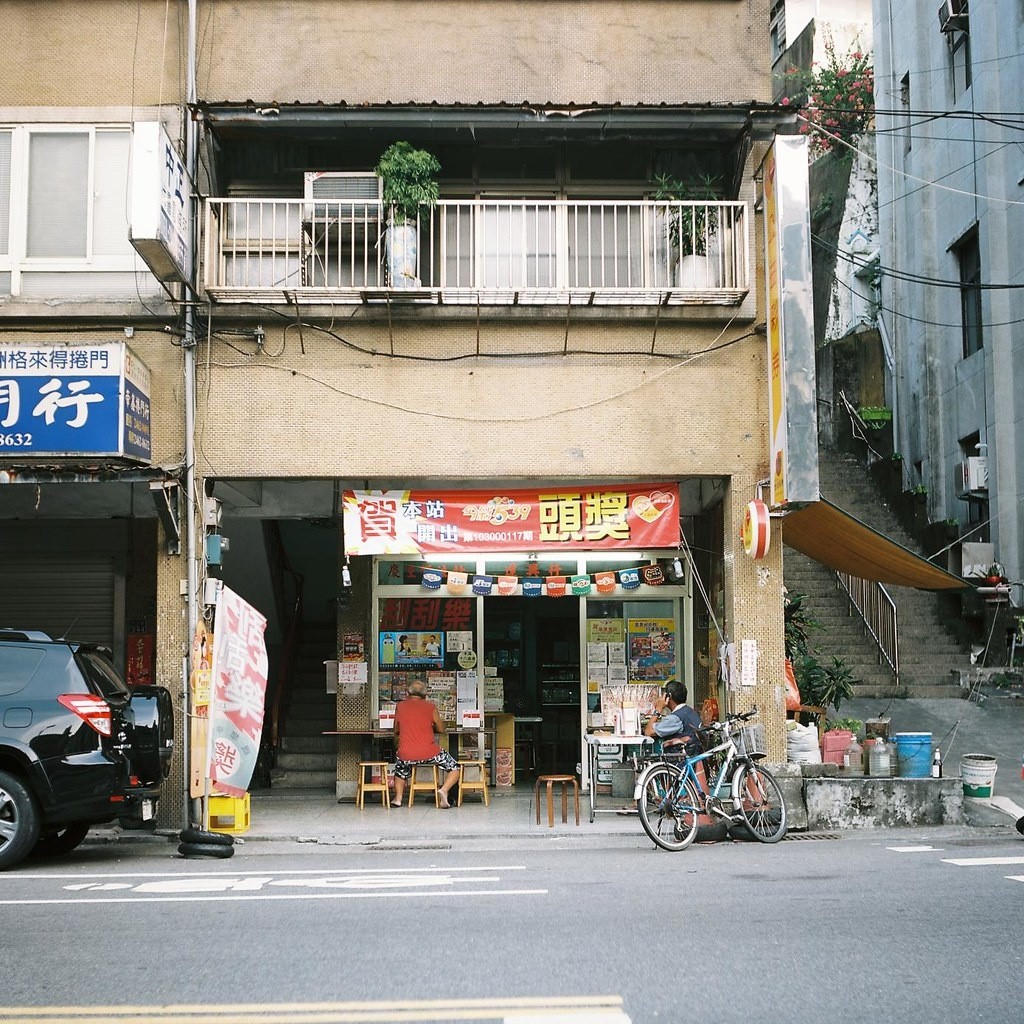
[632, 704, 788, 852]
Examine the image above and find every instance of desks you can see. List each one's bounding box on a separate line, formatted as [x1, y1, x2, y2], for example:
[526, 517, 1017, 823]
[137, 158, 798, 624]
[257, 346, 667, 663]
[584, 735, 655, 822]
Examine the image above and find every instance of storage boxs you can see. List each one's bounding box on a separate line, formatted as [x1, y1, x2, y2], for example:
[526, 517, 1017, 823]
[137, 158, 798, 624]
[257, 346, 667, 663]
[208, 791, 250, 833]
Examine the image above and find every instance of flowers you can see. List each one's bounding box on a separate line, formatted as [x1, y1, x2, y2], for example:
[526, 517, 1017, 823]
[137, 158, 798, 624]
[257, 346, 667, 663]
[859, 405, 887, 411]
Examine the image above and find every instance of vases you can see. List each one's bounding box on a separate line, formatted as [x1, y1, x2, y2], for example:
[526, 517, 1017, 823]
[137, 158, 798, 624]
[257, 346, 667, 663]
[862, 411, 892, 420]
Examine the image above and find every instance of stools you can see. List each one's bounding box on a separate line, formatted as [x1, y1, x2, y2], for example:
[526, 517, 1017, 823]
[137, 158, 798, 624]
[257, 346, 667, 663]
[456, 760, 489, 807]
[536, 775, 579, 828]
[408, 763, 440, 808]
[355, 761, 389, 810]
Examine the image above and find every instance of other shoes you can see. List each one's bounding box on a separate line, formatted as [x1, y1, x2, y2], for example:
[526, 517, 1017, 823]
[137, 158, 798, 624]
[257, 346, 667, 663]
[617, 806, 639, 817]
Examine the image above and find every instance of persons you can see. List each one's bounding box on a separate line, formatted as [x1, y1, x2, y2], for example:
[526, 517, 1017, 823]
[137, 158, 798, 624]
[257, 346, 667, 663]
[424, 635, 439, 655]
[616, 680, 700, 816]
[391, 681, 460, 808]
[399, 636, 411, 652]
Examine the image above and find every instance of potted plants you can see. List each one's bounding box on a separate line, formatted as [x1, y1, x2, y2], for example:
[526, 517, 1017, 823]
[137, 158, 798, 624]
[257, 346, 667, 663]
[374, 142, 442, 287]
[890, 453, 904, 469]
[942, 519, 959, 539]
[649, 173, 724, 287]
[911, 484, 928, 503]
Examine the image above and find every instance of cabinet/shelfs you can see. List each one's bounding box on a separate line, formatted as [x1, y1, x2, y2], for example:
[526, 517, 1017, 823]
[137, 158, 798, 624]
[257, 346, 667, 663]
[536, 663, 580, 705]
[484, 611, 526, 703]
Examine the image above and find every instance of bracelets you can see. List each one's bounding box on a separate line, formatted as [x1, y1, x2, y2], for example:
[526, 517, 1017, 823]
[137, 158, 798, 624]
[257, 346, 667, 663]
[654, 710, 661, 718]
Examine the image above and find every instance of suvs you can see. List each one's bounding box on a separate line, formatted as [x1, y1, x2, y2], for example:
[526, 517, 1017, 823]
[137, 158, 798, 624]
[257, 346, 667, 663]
[0, 626, 175, 870]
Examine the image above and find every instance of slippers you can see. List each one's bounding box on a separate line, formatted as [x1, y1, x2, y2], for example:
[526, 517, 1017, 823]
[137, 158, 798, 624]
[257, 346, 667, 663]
[390, 802, 397, 808]
[443, 804, 453, 808]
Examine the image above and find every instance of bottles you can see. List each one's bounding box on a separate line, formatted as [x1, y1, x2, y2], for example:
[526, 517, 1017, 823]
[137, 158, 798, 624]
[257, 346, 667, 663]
[932, 748, 942, 778]
[674, 557, 684, 578]
[843, 733, 896, 776]
[342, 566, 351, 587]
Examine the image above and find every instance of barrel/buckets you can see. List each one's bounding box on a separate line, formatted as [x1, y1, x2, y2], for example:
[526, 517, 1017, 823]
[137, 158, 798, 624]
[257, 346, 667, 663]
[959, 753, 998, 803]
[895, 732, 932, 778]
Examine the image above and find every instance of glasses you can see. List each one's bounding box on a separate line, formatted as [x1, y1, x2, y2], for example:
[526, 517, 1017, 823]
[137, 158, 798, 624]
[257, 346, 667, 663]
[661, 687, 667, 694]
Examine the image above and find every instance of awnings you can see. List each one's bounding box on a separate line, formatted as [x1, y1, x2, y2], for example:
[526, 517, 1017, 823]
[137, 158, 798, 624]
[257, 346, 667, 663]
[781, 497, 979, 591]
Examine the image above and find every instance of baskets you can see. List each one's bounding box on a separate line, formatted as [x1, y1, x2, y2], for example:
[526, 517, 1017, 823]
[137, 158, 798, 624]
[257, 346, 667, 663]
[720, 723, 767, 763]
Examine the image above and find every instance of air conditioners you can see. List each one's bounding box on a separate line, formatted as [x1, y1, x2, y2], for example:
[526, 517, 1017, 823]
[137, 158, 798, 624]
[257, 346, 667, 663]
[304, 172, 384, 243]
[953, 455, 988, 497]
